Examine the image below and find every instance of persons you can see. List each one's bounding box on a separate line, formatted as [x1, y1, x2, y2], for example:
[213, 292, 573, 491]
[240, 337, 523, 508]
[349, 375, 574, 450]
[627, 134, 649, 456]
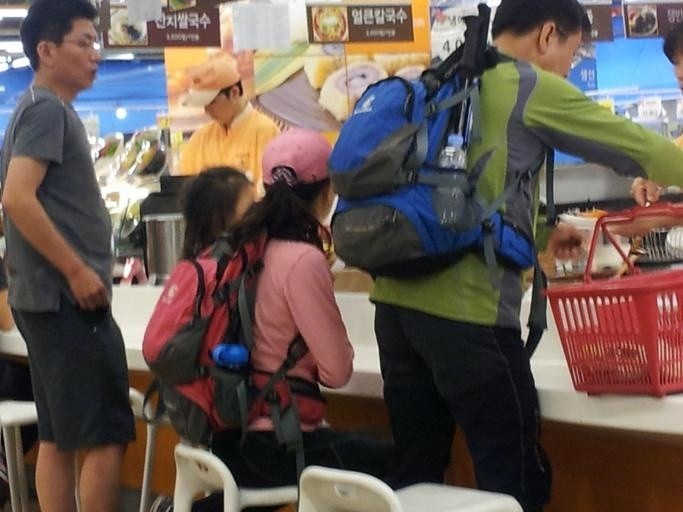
[0, 2, 137, 510]
[365, 3, 683, 511]
[207, 129, 392, 484]
[629, 19, 683, 205]
[148, 164, 262, 512]
[175, 56, 282, 196]
[0, 262, 39, 502]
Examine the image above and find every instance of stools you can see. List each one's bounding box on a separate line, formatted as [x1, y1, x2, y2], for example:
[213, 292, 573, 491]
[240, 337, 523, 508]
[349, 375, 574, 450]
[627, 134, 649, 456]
[1, 383, 525, 512]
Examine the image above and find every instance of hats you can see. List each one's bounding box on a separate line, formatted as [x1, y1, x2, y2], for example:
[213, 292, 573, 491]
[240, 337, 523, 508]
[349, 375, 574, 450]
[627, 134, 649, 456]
[263, 127, 332, 191]
[181, 54, 244, 105]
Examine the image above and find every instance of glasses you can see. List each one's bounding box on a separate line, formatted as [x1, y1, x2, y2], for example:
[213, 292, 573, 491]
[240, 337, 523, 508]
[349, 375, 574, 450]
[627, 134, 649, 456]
[40, 34, 103, 52]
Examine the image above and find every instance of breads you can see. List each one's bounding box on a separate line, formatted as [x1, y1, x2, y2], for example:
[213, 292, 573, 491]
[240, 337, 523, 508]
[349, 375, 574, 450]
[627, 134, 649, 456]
[303, 44, 430, 122]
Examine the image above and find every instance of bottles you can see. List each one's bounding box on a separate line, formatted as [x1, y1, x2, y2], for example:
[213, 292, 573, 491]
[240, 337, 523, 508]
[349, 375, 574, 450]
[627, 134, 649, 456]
[435, 132, 468, 225]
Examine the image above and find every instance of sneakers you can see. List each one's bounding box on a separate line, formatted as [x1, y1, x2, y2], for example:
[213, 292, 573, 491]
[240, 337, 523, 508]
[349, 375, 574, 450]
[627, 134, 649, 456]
[150, 495, 170, 511]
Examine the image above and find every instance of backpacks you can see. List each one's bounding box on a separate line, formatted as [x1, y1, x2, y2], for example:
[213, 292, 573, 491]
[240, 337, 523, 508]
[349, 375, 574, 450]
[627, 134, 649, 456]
[141, 238, 336, 446]
[327, 40, 549, 273]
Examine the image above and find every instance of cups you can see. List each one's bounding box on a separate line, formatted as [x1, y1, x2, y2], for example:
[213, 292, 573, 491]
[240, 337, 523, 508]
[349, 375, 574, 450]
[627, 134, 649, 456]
[211, 340, 252, 428]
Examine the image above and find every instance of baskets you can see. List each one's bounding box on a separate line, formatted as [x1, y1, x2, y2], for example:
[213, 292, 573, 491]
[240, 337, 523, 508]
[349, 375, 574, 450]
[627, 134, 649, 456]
[538, 209, 683, 397]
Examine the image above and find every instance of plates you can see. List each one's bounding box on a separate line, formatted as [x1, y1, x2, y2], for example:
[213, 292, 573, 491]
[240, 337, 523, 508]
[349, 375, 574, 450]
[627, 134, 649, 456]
[311, 6, 348, 40]
[627, 4, 657, 35]
